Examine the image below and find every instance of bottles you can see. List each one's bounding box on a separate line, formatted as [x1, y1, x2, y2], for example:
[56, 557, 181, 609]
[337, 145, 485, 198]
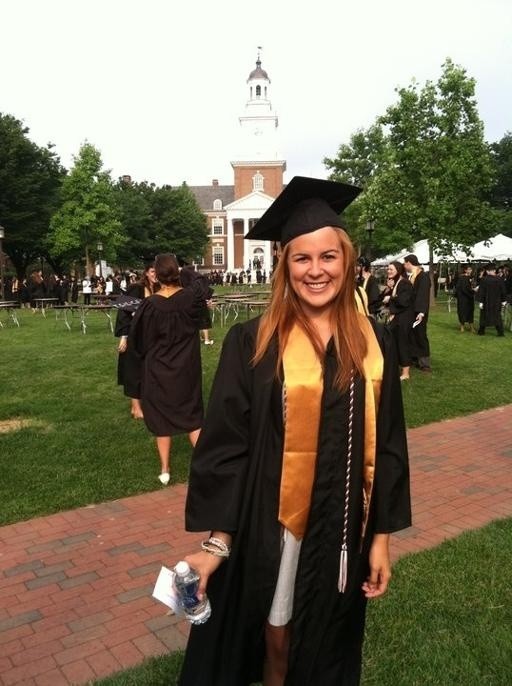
[173, 561, 212, 625]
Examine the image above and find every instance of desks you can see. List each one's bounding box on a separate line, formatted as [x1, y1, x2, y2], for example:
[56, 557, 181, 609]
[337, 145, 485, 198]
[0, 291, 273, 334]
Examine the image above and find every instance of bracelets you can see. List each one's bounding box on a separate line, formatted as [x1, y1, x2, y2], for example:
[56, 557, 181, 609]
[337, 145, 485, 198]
[200, 537, 230, 558]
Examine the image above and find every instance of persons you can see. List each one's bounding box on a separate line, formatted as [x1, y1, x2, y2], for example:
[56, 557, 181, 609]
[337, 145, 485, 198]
[115, 255, 213, 485]
[203, 258, 262, 285]
[177, 175, 412, 686]
[23, 267, 138, 308]
[352, 258, 511, 380]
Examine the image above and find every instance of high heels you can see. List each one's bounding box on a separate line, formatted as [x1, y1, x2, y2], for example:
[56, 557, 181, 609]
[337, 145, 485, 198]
[156, 474, 171, 486]
[200, 339, 213, 345]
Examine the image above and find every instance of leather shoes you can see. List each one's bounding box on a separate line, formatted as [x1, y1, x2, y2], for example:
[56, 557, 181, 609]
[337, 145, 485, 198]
[399, 375, 410, 381]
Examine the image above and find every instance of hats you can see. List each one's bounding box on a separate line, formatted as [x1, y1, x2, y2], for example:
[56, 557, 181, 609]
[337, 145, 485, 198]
[243, 177, 363, 249]
